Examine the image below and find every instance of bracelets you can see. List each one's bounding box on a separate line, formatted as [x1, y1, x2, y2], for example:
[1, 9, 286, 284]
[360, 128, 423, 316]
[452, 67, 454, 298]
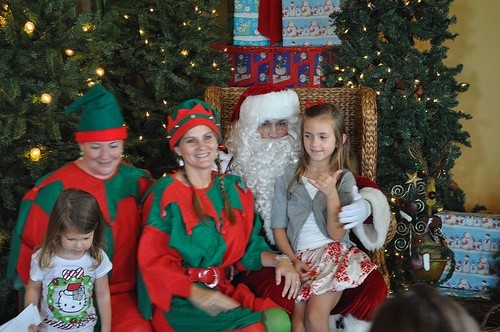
[273, 254, 291, 266]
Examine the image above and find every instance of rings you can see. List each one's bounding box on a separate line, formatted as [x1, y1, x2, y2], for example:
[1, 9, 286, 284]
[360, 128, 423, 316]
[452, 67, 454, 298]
[296, 281, 299, 284]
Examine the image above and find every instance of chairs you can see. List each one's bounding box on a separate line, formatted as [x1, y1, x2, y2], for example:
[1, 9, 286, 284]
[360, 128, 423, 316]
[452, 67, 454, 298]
[204, 85, 391, 297]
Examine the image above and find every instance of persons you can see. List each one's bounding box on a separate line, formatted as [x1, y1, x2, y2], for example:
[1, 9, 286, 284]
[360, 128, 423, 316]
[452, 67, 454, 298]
[4, 81, 158, 332]
[222, 84, 389, 332]
[23, 187, 113, 332]
[137, 100, 302, 332]
[269, 103, 378, 332]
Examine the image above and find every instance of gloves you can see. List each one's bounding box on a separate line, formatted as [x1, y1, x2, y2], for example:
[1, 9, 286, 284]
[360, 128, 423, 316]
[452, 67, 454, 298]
[337, 186, 372, 231]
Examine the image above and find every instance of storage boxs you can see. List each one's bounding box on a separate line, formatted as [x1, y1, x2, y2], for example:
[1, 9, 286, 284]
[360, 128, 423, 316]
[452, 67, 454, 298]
[433, 210, 500, 292]
[210, 0, 345, 87]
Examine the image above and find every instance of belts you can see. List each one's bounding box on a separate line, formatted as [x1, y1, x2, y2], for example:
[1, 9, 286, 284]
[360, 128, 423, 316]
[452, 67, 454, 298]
[182, 265, 231, 288]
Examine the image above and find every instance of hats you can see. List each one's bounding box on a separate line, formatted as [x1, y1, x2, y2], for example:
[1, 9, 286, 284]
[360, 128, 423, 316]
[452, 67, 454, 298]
[64, 84, 127, 141]
[230, 83, 300, 126]
[165, 99, 222, 151]
